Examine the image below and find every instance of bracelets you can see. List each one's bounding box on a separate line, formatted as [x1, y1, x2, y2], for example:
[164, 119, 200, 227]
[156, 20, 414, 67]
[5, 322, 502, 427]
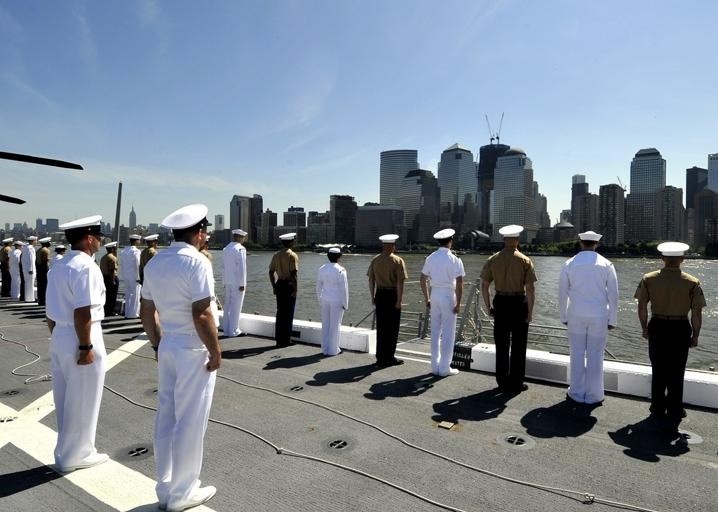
[77, 343, 94, 352]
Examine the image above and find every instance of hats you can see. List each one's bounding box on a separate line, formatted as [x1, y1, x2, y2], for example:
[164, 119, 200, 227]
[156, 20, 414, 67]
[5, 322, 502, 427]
[59, 215, 102, 231]
[279, 232, 296, 240]
[499, 224, 524, 237]
[3, 236, 65, 249]
[329, 248, 341, 253]
[232, 229, 248, 236]
[657, 242, 690, 256]
[379, 234, 399, 243]
[578, 230, 603, 241]
[104, 234, 159, 247]
[433, 228, 455, 239]
[162, 204, 212, 230]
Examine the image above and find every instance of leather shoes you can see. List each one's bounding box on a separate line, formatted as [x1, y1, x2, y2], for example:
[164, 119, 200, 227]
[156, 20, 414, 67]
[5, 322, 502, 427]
[60, 454, 109, 471]
[392, 358, 403, 365]
[168, 486, 216, 512]
[448, 366, 458, 375]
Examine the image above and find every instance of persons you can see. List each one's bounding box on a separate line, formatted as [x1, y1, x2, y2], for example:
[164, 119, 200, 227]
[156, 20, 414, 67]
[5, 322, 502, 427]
[316, 244, 349, 356]
[139, 203, 223, 512]
[2, 227, 160, 322]
[267, 228, 300, 347]
[44, 214, 109, 471]
[557, 231, 619, 408]
[364, 233, 408, 365]
[419, 227, 467, 380]
[479, 221, 538, 393]
[195, 228, 252, 338]
[631, 239, 708, 417]
